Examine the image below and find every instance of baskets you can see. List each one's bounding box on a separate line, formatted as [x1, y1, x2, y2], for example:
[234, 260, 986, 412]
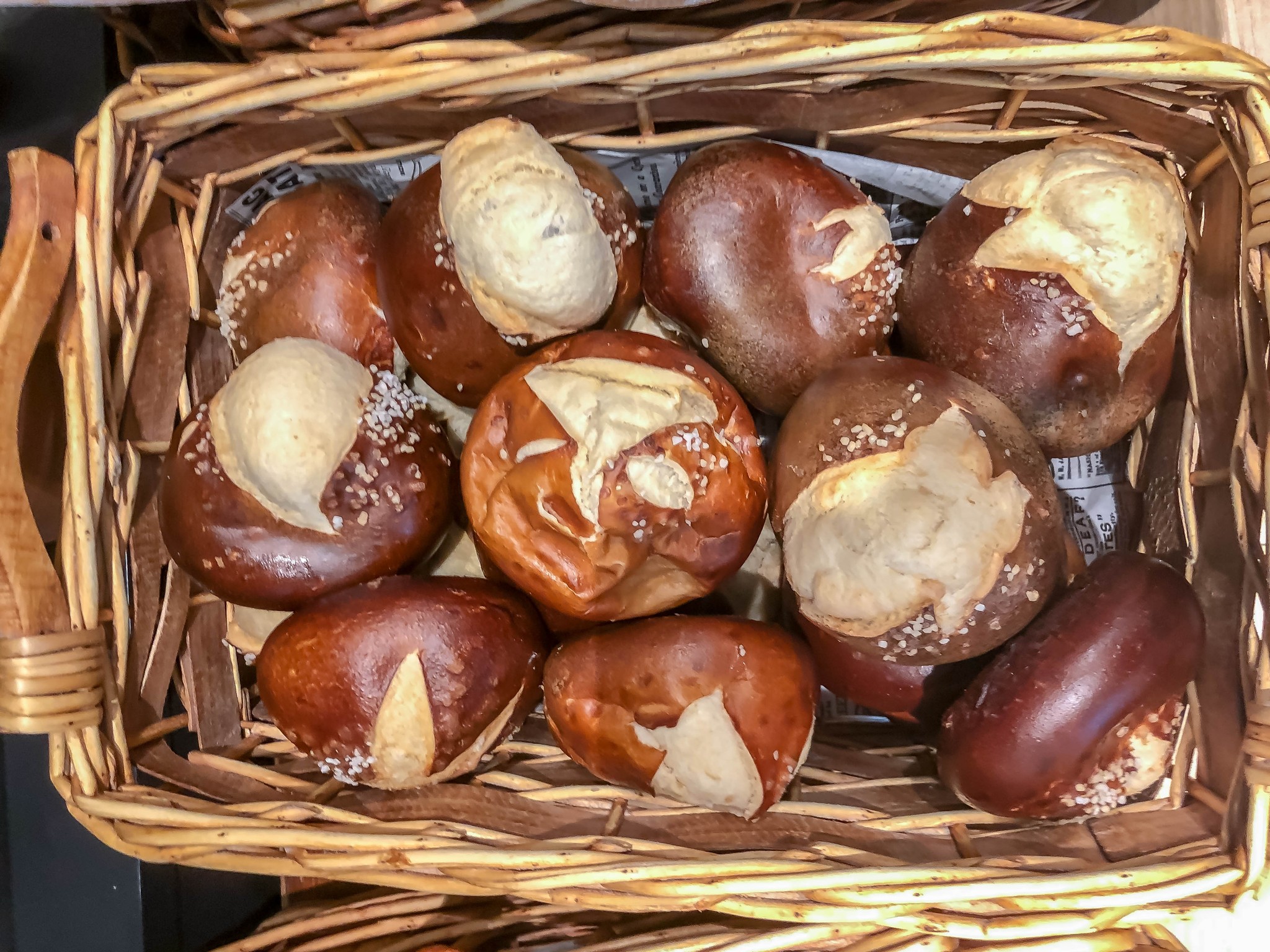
[198, 886, 1270, 952]
[1, 7, 1270, 945]
[192, 0, 1111, 54]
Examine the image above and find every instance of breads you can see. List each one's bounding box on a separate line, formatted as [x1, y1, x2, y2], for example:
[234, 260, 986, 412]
[155, 335, 455, 611]
[898, 132, 1187, 462]
[373, 114, 643, 407]
[541, 613, 819, 821]
[771, 357, 1063, 665]
[642, 137, 902, 416]
[255, 572, 553, 790]
[459, 328, 769, 630]
[776, 531, 1089, 729]
[215, 179, 407, 385]
[932, 547, 1205, 821]
